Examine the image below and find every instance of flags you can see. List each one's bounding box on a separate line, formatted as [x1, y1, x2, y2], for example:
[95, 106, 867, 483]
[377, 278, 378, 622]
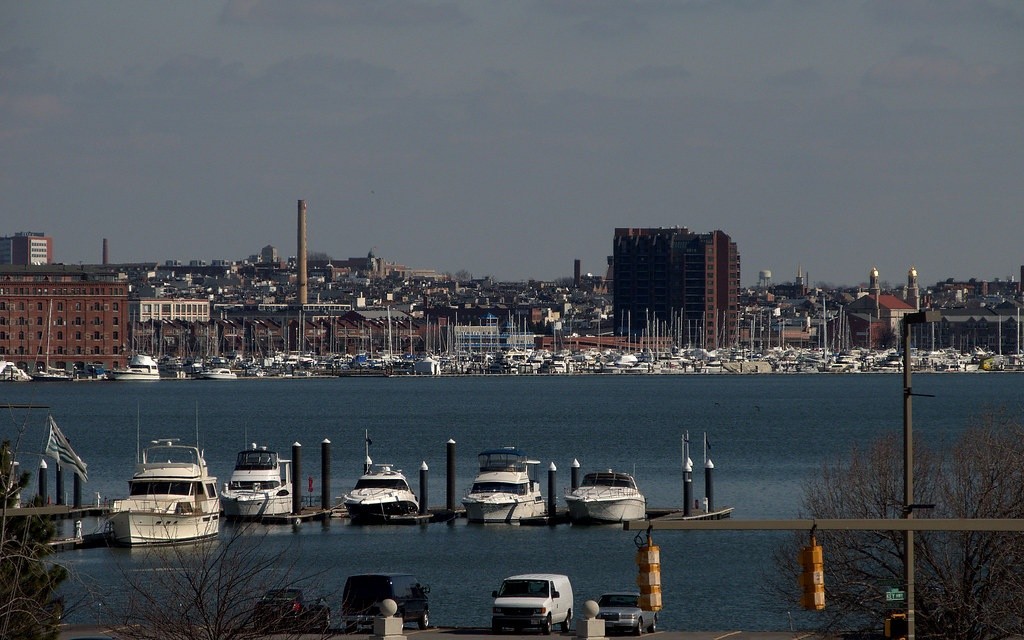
[45, 415, 89, 483]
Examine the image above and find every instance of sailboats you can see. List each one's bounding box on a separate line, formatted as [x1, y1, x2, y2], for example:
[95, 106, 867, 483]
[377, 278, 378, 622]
[1, 297, 1024, 382]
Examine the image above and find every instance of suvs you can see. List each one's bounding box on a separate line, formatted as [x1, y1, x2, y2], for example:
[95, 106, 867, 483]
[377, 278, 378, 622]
[342, 572, 432, 632]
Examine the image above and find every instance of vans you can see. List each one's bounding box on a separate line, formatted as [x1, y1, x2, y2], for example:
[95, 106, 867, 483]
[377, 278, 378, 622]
[491, 574, 574, 635]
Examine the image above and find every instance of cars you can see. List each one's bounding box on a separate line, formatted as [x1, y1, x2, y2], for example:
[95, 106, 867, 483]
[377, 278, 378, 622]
[254, 588, 330, 629]
[595, 591, 660, 634]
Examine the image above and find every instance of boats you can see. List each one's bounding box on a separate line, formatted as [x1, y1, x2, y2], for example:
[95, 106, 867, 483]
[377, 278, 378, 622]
[460, 447, 547, 522]
[220, 449, 293, 520]
[108, 438, 221, 545]
[564, 469, 645, 523]
[343, 463, 419, 524]
